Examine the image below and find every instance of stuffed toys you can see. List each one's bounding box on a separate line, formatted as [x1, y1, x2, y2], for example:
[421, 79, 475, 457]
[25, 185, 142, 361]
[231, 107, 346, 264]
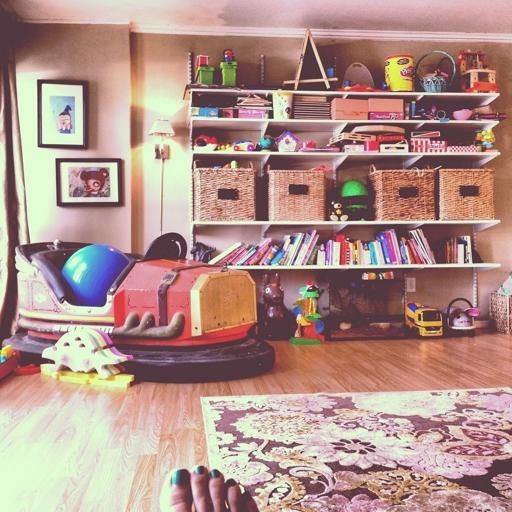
[325, 194, 350, 221]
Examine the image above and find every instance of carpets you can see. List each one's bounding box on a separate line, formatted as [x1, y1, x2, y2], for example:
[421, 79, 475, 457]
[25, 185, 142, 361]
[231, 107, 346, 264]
[200, 386, 512, 512]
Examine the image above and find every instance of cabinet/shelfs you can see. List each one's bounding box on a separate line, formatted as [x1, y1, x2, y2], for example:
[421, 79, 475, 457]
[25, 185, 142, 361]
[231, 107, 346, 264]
[182, 83, 501, 271]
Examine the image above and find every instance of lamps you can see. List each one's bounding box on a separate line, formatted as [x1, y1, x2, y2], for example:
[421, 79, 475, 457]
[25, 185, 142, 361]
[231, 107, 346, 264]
[148, 115, 174, 236]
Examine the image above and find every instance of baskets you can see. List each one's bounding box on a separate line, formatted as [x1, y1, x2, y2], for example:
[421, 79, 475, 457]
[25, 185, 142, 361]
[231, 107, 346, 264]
[488, 291, 512, 334]
[368, 164, 437, 220]
[267, 164, 326, 220]
[438, 166, 496, 220]
[194, 159, 256, 221]
[415, 50, 456, 92]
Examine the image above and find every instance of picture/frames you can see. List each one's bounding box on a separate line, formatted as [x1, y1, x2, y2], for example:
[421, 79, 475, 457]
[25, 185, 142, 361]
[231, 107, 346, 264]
[55, 157, 121, 208]
[36, 78, 89, 149]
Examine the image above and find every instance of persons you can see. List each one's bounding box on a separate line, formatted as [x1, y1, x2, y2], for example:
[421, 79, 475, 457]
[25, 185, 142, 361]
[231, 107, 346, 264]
[158, 464, 260, 512]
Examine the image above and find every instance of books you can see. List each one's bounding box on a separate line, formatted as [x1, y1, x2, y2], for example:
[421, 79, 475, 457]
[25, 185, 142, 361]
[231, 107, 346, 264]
[233, 94, 274, 111]
[292, 94, 331, 120]
[202, 228, 475, 266]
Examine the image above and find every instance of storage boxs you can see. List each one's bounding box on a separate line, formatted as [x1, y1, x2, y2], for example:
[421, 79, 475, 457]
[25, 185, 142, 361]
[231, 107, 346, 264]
[330, 97, 369, 120]
[369, 98, 403, 112]
